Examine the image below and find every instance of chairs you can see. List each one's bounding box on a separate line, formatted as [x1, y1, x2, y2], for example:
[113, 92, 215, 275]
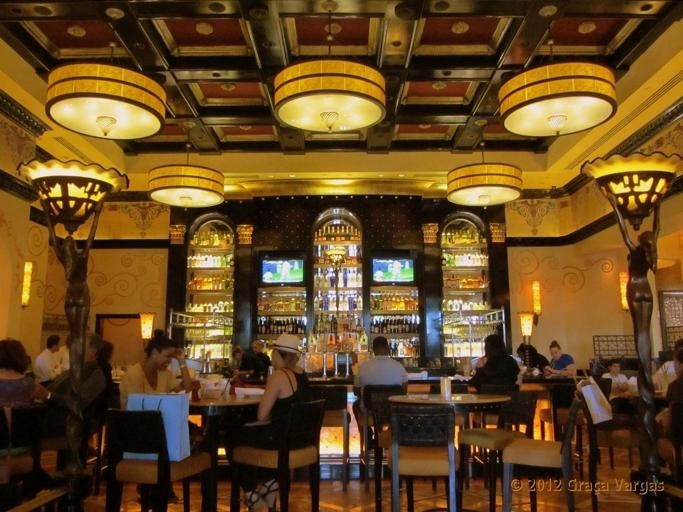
[0, 371, 683, 512]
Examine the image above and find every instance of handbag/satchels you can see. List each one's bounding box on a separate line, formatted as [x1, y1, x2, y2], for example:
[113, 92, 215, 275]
[122, 393, 191, 462]
[576, 376, 613, 425]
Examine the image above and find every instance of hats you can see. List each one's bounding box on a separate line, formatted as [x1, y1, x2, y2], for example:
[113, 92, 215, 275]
[269, 332, 304, 354]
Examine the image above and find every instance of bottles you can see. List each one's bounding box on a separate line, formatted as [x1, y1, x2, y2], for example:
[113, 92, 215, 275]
[312, 268, 362, 289]
[184, 316, 233, 337]
[442, 315, 488, 337]
[256, 340, 275, 360]
[440, 248, 487, 268]
[255, 316, 305, 337]
[385, 337, 419, 359]
[185, 271, 234, 292]
[256, 289, 306, 313]
[308, 334, 369, 356]
[442, 339, 486, 357]
[369, 315, 419, 335]
[312, 290, 362, 312]
[311, 244, 362, 266]
[440, 271, 486, 289]
[187, 300, 234, 313]
[188, 230, 233, 247]
[314, 225, 361, 242]
[368, 285, 419, 313]
[312, 313, 363, 334]
[185, 252, 234, 269]
[185, 342, 232, 363]
[441, 295, 486, 310]
[440, 228, 486, 247]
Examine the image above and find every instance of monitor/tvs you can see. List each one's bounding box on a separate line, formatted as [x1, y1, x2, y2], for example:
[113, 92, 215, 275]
[253, 252, 308, 287]
[368, 251, 418, 286]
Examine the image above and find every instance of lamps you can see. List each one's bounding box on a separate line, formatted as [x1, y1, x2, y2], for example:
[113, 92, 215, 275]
[270, 1, 389, 136]
[519, 311, 537, 371]
[145, 119, 224, 210]
[618, 270, 630, 313]
[530, 280, 542, 318]
[19, 260, 34, 310]
[446, 120, 523, 209]
[45, 8, 168, 141]
[139, 311, 155, 350]
[499, 6, 619, 137]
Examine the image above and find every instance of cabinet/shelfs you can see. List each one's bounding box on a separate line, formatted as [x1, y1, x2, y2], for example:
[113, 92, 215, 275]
[254, 287, 308, 338]
[370, 286, 421, 358]
[438, 217, 491, 359]
[188, 220, 233, 366]
[312, 212, 362, 353]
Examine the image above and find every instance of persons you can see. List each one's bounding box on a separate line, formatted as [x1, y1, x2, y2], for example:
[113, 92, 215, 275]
[350, 334, 409, 452]
[464, 334, 519, 434]
[605, 191, 664, 360]
[517, 341, 683, 512]
[225, 334, 314, 512]
[372, 258, 415, 282]
[0, 328, 268, 509]
[262, 260, 304, 283]
[38, 197, 105, 375]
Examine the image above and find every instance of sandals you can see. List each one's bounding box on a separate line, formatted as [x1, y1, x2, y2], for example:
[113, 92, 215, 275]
[259, 480, 280, 511]
[244, 491, 268, 512]
